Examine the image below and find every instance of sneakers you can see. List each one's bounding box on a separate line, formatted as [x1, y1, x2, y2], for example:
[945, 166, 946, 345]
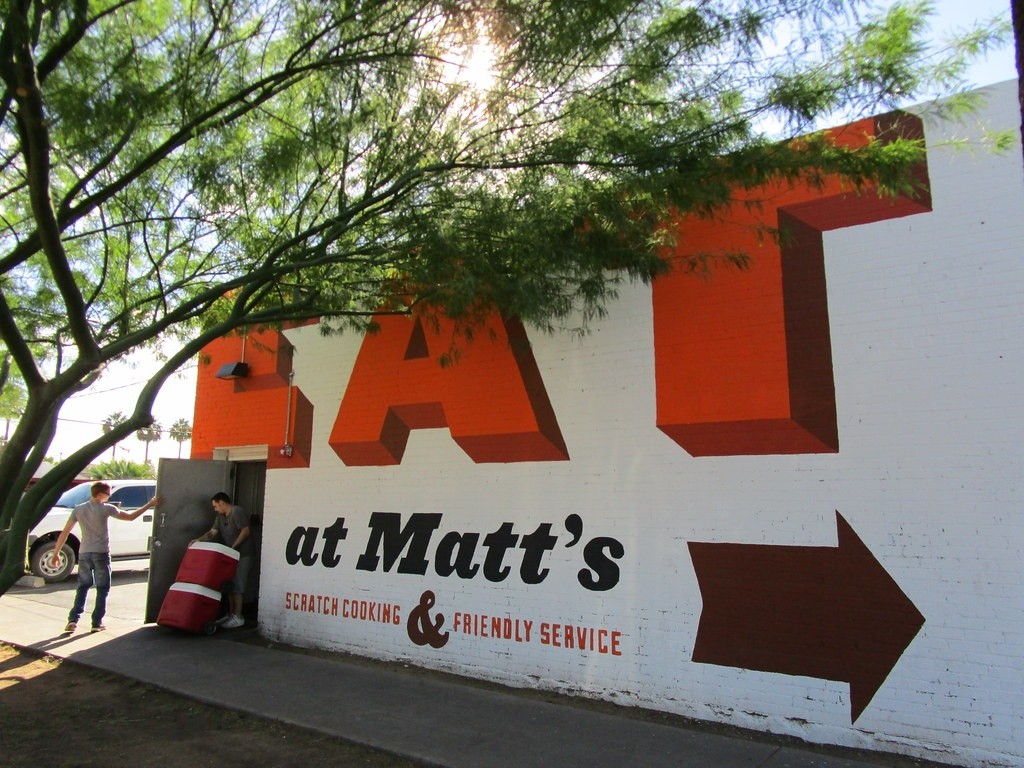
[91, 624, 106, 632]
[65, 622, 77, 632]
[221, 613, 244, 628]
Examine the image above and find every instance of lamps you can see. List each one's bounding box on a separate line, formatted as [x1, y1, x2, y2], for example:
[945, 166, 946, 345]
[216, 362, 249, 379]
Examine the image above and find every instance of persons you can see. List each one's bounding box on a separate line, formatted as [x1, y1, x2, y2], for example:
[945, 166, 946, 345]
[188, 493, 250, 628]
[52, 482, 164, 633]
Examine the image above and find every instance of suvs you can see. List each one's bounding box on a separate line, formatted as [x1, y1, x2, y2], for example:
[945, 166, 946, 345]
[29, 478, 157, 582]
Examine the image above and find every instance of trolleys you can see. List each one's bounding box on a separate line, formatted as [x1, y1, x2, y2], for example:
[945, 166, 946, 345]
[159, 620, 216, 636]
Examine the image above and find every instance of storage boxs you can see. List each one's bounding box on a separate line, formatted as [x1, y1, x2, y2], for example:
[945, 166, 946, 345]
[156, 582, 222, 633]
[176, 541, 240, 591]
